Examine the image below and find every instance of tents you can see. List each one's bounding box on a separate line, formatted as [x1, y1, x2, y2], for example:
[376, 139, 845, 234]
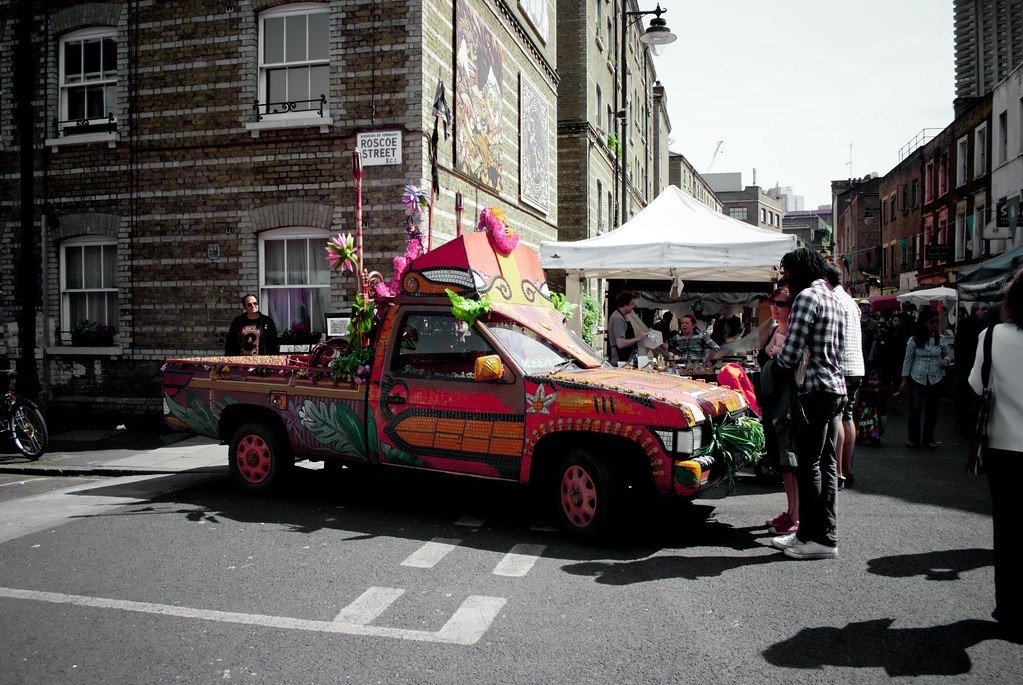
[870, 294, 901, 315]
[956, 243, 1023, 336]
[538, 184, 797, 355]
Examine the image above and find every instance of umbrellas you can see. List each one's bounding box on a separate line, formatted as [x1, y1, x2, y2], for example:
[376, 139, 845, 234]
[896, 284, 958, 333]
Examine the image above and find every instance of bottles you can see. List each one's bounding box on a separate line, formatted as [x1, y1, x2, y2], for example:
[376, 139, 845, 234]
[747, 351, 753, 363]
[674, 366, 678, 376]
[646, 349, 653, 364]
[686, 356, 692, 371]
[658, 355, 664, 368]
[715, 364, 720, 374]
[697, 359, 703, 365]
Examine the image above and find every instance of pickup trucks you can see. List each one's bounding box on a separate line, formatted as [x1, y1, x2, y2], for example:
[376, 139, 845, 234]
[160, 231, 780, 536]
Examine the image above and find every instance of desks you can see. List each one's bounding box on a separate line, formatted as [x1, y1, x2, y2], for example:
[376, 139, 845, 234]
[681, 371, 761, 397]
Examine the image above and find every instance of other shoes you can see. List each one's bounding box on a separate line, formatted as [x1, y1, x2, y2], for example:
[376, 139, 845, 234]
[905, 439, 921, 449]
[923, 442, 938, 449]
[843, 472, 855, 488]
[837, 475, 845, 490]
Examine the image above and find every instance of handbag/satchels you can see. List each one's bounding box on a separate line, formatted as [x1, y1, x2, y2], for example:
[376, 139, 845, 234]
[793, 348, 810, 388]
[966, 388, 995, 476]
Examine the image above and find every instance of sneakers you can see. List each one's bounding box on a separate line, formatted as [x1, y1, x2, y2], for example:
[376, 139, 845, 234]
[766, 511, 790, 527]
[769, 517, 800, 534]
[771, 532, 805, 549]
[784, 540, 838, 559]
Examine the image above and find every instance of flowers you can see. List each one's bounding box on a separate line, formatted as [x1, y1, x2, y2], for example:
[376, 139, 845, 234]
[323, 185, 434, 382]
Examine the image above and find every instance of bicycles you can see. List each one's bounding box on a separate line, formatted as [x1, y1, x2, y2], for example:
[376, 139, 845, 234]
[0, 369, 48, 460]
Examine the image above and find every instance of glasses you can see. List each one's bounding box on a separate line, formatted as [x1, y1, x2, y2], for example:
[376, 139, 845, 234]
[246, 302, 258, 308]
[770, 299, 789, 308]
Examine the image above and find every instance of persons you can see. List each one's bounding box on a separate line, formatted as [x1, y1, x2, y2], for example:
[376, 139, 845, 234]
[825, 262, 865, 489]
[954, 302, 990, 373]
[858, 300, 881, 364]
[756, 286, 799, 534]
[862, 368, 904, 447]
[665, 314, 720, 363]
[776, 247, 849, 559]
[652, 312, 679, 343]
[606, 290, 650, 369]
[967, 269, 1023, 625]
[877, 314, 902, 413]
[225, 294, 279, 356]
[900, 310, 953, 449]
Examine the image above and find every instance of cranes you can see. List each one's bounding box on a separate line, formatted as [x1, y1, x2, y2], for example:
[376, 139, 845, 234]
[701, 141, 724, 174]
